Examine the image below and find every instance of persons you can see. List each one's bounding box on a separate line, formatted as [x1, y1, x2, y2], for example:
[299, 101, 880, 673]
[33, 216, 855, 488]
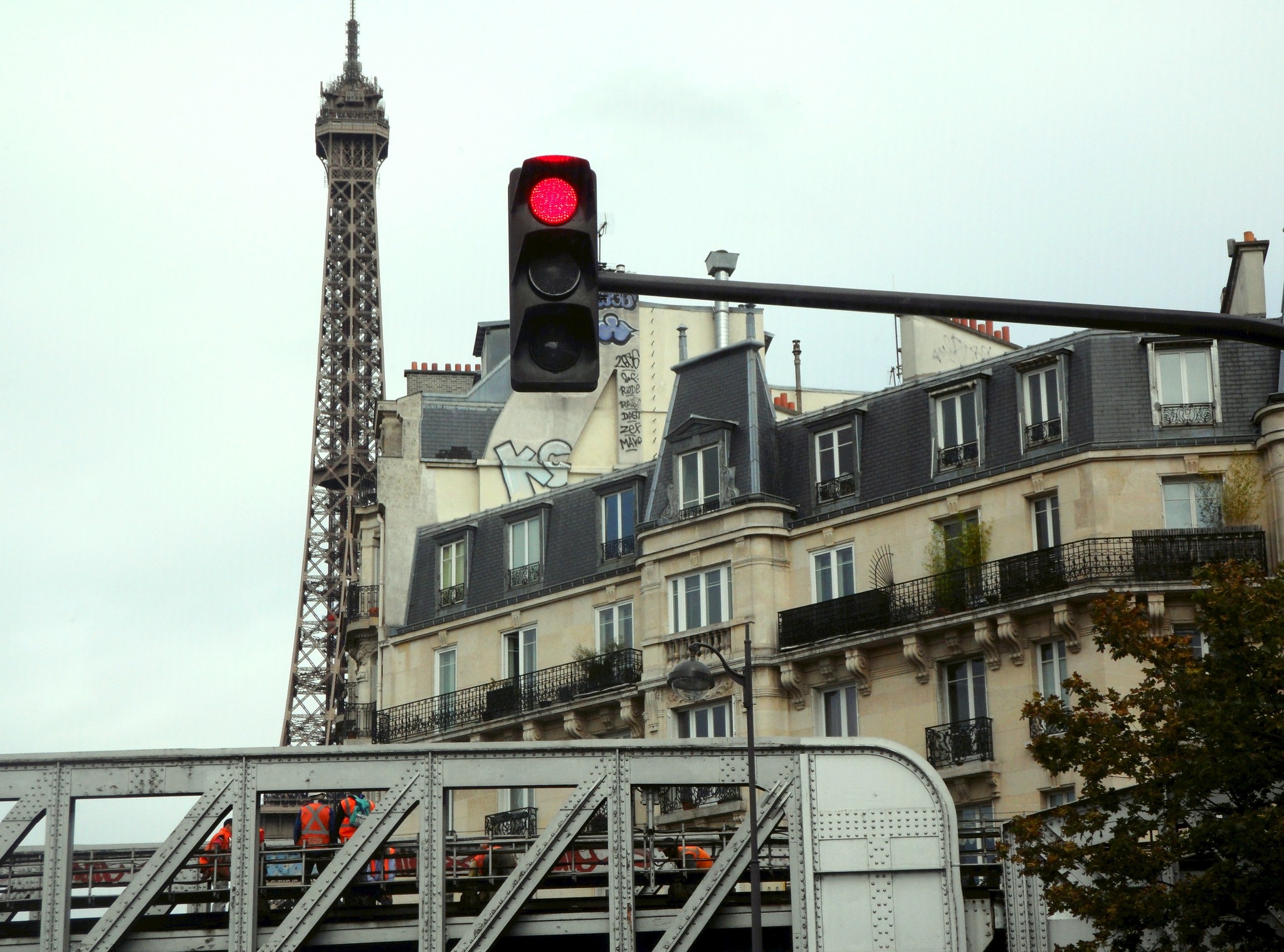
[657, 837, 716, 909]
[293, 791, 335, 886]
[460, 840, 531, 914]
[335, 783, 398, 908]
[197, 818, 268, 904]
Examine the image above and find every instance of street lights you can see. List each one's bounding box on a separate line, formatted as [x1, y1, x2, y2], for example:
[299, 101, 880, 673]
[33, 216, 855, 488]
[667, 625, 763, 952]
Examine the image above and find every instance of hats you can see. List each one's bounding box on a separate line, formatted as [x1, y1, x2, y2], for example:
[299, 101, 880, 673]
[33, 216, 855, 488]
[658, 837, 675, 851]
[308, 792, 326, 796]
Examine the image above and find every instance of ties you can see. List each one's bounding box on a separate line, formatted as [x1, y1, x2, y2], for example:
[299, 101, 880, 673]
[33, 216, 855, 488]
[374, 862, 380, 880]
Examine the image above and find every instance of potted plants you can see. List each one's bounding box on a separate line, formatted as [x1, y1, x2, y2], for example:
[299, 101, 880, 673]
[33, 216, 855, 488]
[570, 634, 624, 691]
[922, 509, 997, 616]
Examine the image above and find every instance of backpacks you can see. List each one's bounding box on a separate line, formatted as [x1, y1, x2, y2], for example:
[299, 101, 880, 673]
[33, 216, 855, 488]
[349, 793, 370, 827]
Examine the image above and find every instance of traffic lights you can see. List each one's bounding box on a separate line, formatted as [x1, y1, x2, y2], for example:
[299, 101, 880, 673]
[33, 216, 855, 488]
[506, 154, 600, 395]
